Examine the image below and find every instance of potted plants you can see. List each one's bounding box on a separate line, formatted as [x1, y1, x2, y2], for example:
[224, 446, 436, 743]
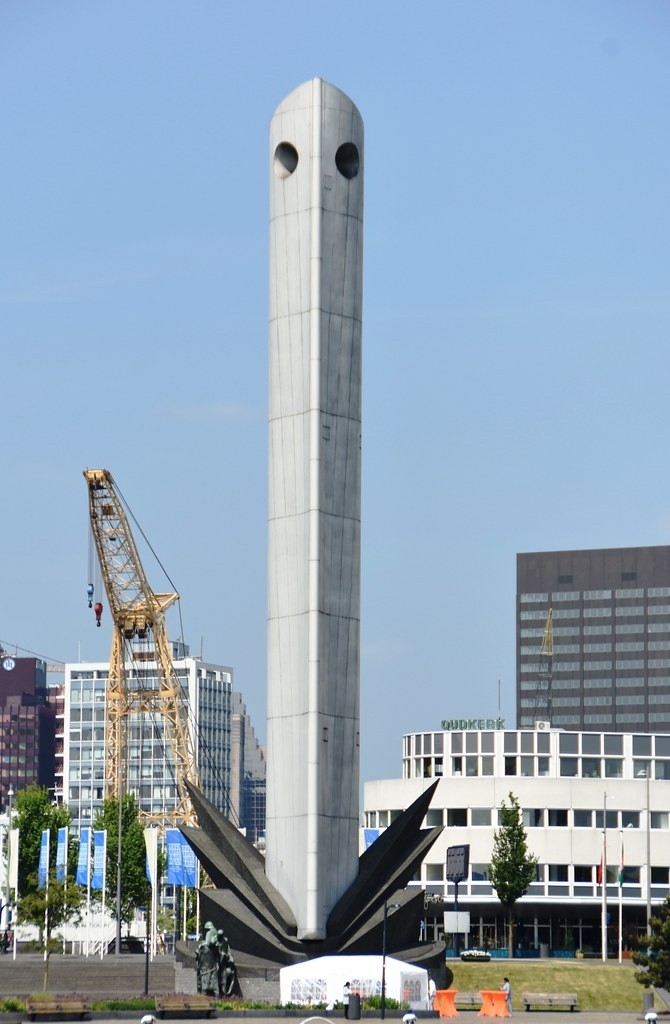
[615, 919, 641, 959]
[575, 949, 584, 959]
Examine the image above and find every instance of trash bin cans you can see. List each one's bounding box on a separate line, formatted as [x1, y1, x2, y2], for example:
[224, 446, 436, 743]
[643, 992, 654, 1012]
[540, 945, 549, 958]
[348, 994, 360, 1019]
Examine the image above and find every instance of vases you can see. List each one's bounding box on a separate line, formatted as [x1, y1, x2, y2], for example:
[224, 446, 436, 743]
[461, 954, 490, 962]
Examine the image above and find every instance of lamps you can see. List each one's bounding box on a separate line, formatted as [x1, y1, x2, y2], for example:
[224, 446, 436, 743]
[323, 727, 329, 743]
[356, 732, 360, 747]
[534, 721, 550, 731]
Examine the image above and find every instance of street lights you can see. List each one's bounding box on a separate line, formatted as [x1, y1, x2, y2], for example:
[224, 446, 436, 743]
[382, 903, 400, 1018]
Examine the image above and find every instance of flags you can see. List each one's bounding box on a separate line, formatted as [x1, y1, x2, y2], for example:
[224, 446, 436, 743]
[165, 829, 199, 889]
[56, 828, 65, 883]
[76, 829, 89, 886]
[618, 846, 624, 887]
[143, 828, 156, 885]
[598, 847, 606, 885]
[38, 831, 48, 888]
[91, 832, 104, 888]
[7, 829, 17, 888]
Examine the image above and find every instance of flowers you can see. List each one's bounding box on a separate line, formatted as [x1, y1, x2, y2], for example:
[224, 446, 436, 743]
[460, 950, 492, 956]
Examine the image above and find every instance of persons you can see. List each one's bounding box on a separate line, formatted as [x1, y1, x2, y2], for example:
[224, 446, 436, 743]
[343, 982, 352, 1019]
[500, 977, 513, 1014]
[428, 976, 436, 1011]
[198, 921, 236, 998]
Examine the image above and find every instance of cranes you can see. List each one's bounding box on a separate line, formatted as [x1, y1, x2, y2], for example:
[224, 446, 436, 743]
[83, 466, 204, 830]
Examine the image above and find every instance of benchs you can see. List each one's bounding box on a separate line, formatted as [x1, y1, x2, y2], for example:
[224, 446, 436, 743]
[452, 991, 485, 1006]
[522, 991, 580, 1013]
[155, 994, 217, 1020]
[25, 996, 93, 1022]
